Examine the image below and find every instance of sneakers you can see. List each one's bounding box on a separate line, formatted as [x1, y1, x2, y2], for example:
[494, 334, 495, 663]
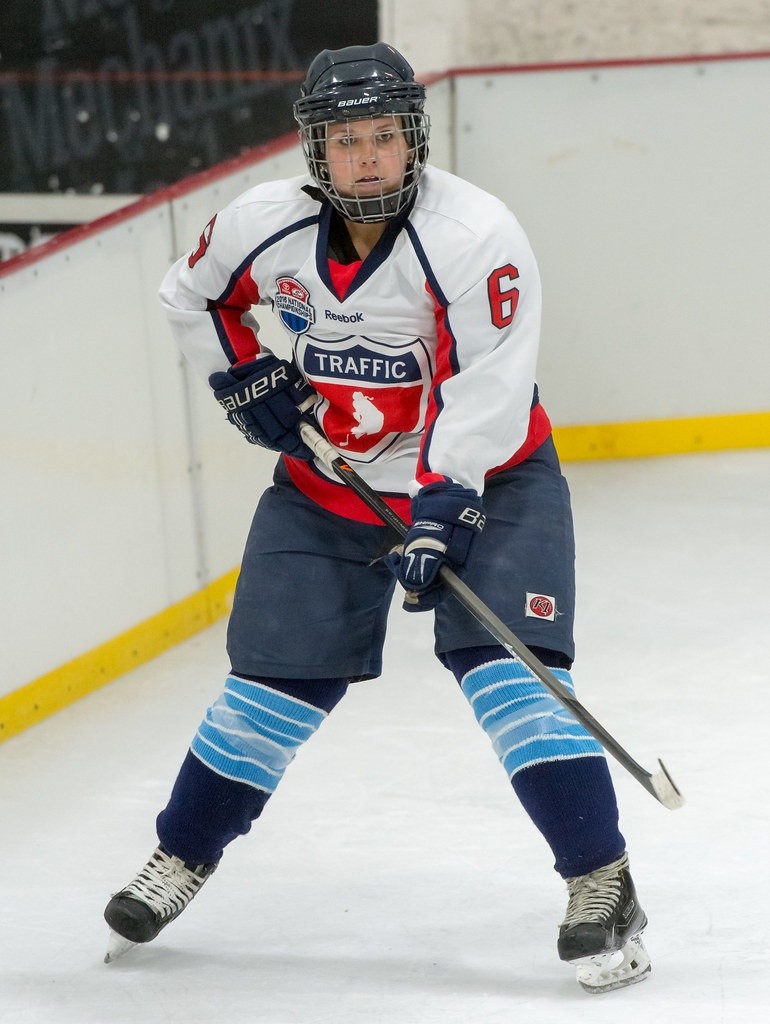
[556, 850, 651, 994]
[104, 838, 220, 964]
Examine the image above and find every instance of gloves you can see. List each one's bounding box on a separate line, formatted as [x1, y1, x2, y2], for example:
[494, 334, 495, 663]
[208, 355, 318, 461]
[384, 480, 486, 612]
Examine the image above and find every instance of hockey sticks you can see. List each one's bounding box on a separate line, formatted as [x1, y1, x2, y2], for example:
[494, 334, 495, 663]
[298, 420, 687, 811]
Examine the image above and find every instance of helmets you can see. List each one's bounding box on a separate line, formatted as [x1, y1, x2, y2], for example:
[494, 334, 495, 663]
[293, 42, 432, 224]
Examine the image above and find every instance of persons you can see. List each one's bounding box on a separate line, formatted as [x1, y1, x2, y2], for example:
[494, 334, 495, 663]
[104, 42, 650, 994]
